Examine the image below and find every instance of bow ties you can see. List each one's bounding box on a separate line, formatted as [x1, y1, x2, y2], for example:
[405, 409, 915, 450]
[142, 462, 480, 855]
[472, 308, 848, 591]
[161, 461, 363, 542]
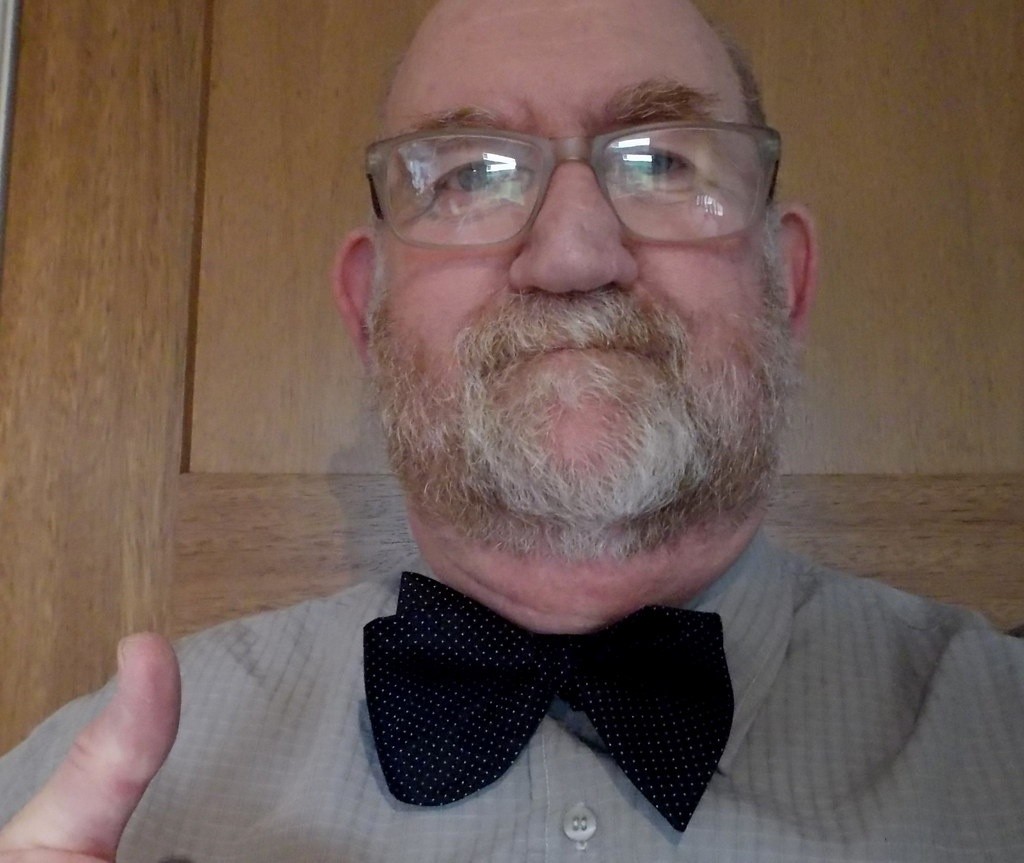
[362, 569, 735, 834]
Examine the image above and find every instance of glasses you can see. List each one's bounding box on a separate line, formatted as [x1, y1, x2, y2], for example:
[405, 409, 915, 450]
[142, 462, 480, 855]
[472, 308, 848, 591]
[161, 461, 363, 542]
[365, 121, 781, 249]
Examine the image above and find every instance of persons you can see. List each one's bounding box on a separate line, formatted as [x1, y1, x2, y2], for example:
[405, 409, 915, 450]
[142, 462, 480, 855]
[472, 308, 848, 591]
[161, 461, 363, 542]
[1, 0, 1024, 863]
